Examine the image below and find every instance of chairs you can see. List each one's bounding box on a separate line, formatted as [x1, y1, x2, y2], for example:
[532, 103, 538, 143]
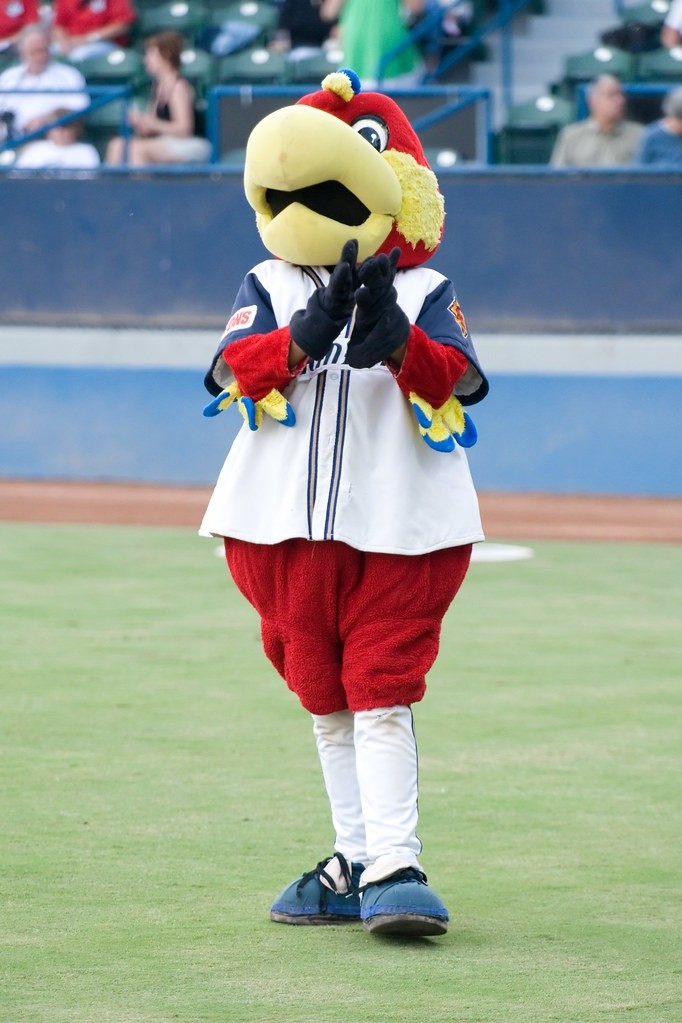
[0, 0, 546, 163]
[497, 0, 682, 165]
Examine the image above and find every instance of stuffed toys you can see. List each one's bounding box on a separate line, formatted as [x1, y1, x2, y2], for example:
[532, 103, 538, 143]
[202, 67, 489, 935]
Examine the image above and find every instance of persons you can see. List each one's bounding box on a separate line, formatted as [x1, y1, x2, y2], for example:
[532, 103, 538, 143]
[551, 72, 682, 167]
[660, 0, 682, 62]
[0, 0, 138, 179]
[403, 0, 487, 85]
[269, 0, 346, 84]
[105, 31, 214, 180]
